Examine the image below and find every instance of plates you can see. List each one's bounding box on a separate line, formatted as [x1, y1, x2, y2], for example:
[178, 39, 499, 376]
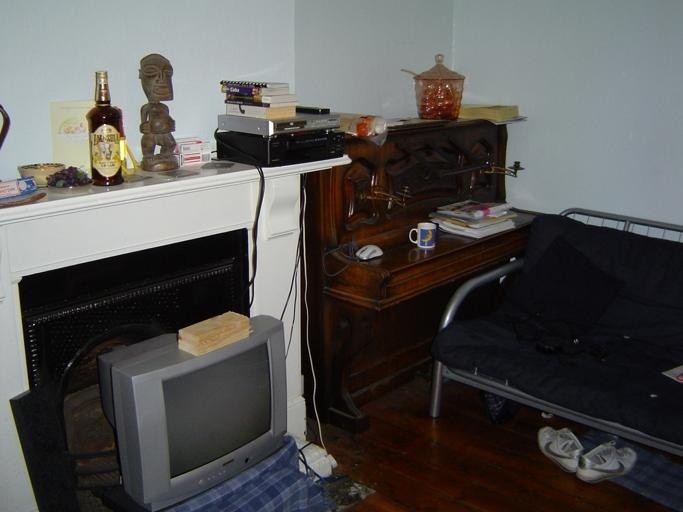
[49, 179, 95, 194]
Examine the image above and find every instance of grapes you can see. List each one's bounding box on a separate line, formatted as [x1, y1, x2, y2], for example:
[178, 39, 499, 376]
[47, 167, 92, 186]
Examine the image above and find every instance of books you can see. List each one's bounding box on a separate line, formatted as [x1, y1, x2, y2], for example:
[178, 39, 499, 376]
[438, 200, 512, 221]
[219, 80, 299, 119]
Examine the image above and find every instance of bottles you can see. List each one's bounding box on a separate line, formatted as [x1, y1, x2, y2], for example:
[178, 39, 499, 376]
[89, 71, 124, 187]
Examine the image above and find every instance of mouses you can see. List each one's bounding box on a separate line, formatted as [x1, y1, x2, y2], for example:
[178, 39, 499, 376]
[355, 244, 384, 260]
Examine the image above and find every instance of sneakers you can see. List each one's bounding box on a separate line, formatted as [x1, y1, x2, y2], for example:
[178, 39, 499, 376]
[538, 426, 585, 474]
[577, 442, 637, 484]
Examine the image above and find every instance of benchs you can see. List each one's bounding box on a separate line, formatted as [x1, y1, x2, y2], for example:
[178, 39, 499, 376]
[429, 209, 681, 459]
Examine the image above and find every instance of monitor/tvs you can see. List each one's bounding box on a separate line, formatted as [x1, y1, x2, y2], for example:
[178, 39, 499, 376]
[96, 314, 288, 512]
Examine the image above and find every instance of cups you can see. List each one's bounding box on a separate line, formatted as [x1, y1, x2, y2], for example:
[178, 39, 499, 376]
[409, 223, 437, 249]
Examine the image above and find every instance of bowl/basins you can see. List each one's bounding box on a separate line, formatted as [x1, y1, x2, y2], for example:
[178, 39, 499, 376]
[18, 163, 65, 187]
